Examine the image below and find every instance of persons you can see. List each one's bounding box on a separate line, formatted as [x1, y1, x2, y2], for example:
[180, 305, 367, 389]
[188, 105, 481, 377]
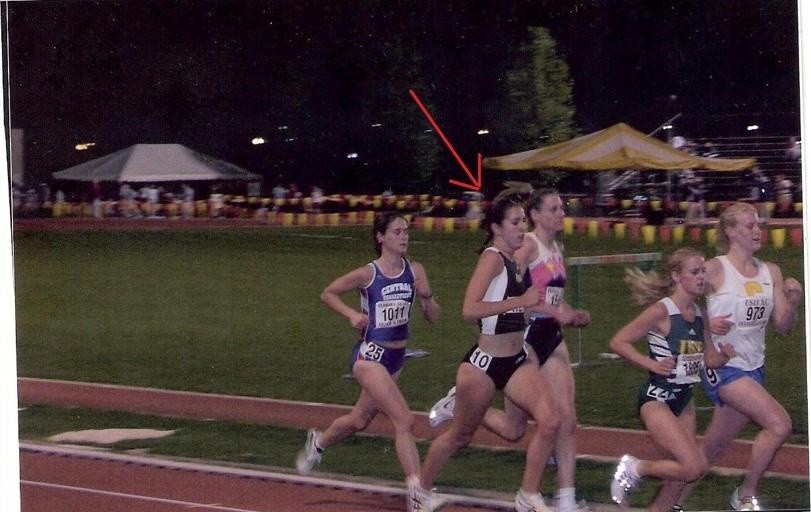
[12, 133, 803, 222]
[292, 207, 454, 510]
[674, 200, 804, 511]
[420, 196, 565, 512]
[608, 245, 739, 511]
[428, 186, 593, 511]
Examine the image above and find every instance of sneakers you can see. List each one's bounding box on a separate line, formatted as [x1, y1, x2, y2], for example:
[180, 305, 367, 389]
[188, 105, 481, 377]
[514, 488, 551, 512]
[429, 387, 456, 427]
[610, 453, 641, 504]
[294, 428, 325, 477]
[729, 484, 761, 511]
[408, 485, 448, 512]
